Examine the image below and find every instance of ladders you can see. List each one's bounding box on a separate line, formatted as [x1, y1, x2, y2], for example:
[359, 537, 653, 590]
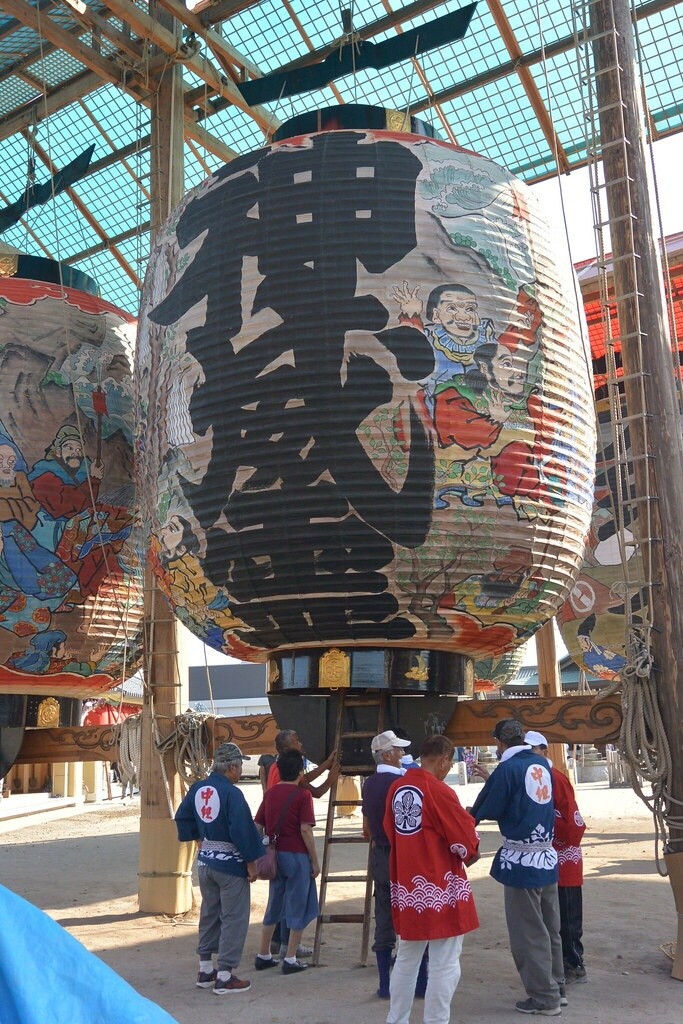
[312, 688, 386, 967]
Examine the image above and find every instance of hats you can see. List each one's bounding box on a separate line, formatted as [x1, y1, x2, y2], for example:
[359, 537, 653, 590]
[213, 743, 251, 763]
[523, 730, 548, 750]
[491, 720, 525, 745]
[371, 730, 411, 754]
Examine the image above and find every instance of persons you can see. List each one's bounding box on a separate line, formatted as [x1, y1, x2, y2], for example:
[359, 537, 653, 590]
[174, 743, 257, 995]
[253, 750, 320, 975]
[393, 728, 420, 770]
[463, 746, 491, 782]
[267, 728, 342, 957]
[470, 718, 568, 1015]
[361, 730, 429, 1000]
[523, 730, 586, 984]
[382, 735, 481, 1024]
[113, 762, 135, 798]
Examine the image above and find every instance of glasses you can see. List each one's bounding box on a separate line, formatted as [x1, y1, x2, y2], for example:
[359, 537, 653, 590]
[388, 747, 404, 754]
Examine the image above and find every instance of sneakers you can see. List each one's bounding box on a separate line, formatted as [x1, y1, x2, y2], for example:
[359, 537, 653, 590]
[212, 973, 251, 994]
[196, 968, 218, 989]
[560, 986, 568, 1006]
[515, 998, 562, 1016]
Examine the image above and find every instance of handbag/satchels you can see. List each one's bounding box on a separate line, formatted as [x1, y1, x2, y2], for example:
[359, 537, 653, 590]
[254, 835, 276, 880]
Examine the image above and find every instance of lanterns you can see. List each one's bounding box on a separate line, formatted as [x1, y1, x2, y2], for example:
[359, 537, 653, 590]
[135, 103, 597, 697]
[554, 246, 682, 677]
[0, 252, 138, 702]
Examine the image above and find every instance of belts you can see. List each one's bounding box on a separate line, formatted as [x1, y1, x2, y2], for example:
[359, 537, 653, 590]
[201, 841, 235, 852]
[503, 839, 552, 851]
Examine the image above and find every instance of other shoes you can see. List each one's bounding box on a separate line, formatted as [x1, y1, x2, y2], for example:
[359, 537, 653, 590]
[563, 961, 588, 984]
[255, 957, 280, 971]
[270, 942, 280, 954]
[282, 960, 308, 974]
[279, 944, 314, 958]
